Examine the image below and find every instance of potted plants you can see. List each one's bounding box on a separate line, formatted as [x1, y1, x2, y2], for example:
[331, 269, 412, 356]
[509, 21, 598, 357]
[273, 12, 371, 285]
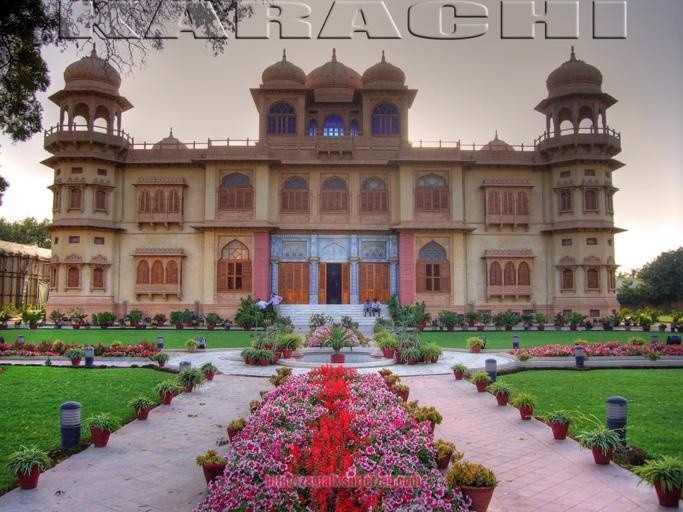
[414, 310, 683, 332]
[318, 327, 355, 363]
[0, 311, 257, 330]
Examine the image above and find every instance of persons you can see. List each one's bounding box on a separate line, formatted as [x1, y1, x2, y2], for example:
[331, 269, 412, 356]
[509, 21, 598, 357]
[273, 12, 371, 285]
[256, 292, 282, 320]
[372, 299, 381, 317]
[363, 299, 373, 317]
